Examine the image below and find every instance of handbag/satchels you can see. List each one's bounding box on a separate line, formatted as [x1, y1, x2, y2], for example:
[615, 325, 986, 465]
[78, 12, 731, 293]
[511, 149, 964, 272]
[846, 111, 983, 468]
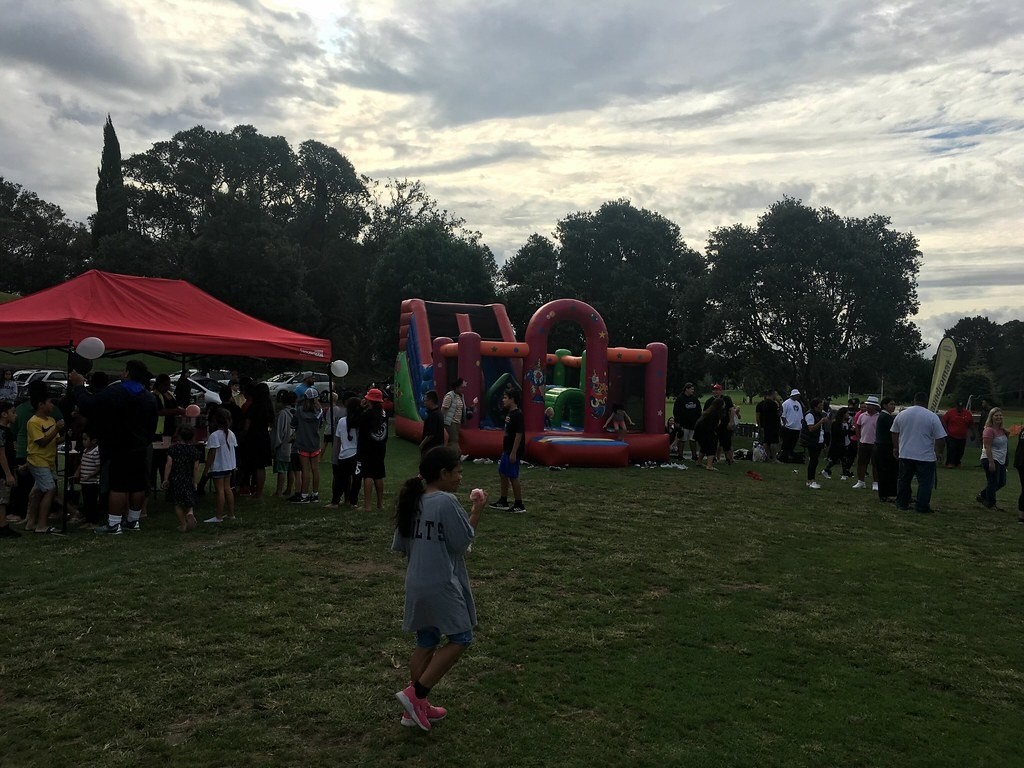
[287, 432, 296, 443]
[670, 433, 680, 451]
[290, 451, 302, 471]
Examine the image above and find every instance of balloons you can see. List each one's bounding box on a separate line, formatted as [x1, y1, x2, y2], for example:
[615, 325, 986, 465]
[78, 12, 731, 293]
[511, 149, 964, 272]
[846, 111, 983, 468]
[76, 336, 104, 358]
[330, 360, 348, 377]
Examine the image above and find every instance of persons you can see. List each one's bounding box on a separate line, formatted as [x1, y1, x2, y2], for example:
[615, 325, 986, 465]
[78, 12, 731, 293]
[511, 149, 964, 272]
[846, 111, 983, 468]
[799, 398, 829, 489]
[488, 386, 528, 513]
[779, 387, 804, 463]
[390, 447, 487, 733]
[889, 391, 948, 514]
[418, 389, 445, 465]
[440, 377, 478, 448]
[666, 381, 741, 471]
[820, 395, 882, 492]
[869, 396, 900, 503]
[1013, 426, 1024, 525]
[602, 403, 635, 433]
[756, 390, 784, 464]
[978, 398, 995, 460]
[940, 398, 973, 470]
[544, 406, 555, 430]
[974, 407, 1008, 512]
[0, 360, 394, 534]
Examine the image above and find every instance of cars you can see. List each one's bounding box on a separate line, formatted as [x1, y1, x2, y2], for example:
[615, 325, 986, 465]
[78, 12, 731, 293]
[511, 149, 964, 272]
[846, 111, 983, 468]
[14, 382, 69, 407]
[168, 369, 232, 386]
[169, 377, 222, 406]
[13, 371, 33, 383]
[24, 370, 90, 390]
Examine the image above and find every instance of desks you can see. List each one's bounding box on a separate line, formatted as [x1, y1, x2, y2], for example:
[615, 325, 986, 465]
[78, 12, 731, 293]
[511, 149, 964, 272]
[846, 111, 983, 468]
[55, 441, 210, 502]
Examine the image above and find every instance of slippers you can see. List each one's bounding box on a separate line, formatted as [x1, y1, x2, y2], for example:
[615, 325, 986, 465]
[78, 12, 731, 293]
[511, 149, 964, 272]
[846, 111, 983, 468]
[24, 521, 61, 533]
[203, 516, 223, 522]
[223, 514, 235, 519]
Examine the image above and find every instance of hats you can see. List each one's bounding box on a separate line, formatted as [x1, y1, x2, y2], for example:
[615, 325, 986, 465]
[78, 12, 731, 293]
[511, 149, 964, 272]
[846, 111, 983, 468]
[790, 389, 800, 397]
[865, 396, 882, 408]
[301, 371, 315, 380]
[365, 389, 384, 402]
[304, 387, 318, 398]
[714, 384, 722, 390]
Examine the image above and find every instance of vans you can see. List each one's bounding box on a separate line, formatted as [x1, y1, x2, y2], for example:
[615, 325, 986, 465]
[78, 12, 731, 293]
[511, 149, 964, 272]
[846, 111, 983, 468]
[260, 372, 334, 403]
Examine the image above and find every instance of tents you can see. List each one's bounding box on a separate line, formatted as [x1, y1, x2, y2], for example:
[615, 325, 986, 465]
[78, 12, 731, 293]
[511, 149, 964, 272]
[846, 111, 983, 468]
[0, 268, 336, 446]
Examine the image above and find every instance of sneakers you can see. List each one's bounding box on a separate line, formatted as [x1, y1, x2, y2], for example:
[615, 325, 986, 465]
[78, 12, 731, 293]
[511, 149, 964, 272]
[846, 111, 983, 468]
[634, 460, 688, 470]
[806, 469, 879, 491]
[401, 703, 447, 726]
[288, 494, 310, 503]
[396, 686, 431, 731]
[309, 494, 319, 502]
[505, 504, 526, 513]
[460, 454, 469, 462]
[94, 523, 123, 535]
[489, 501, 510, 510]
[121, 519, 141, 530]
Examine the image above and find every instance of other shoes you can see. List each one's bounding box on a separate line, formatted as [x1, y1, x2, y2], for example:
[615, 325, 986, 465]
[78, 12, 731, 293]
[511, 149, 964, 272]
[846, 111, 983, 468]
[1017, 517, 1024, 525]
[177, 513, 195, 533]
[880, 462, 997, 514]
[678, 453, 734, 472]
[323, 504, 339, 509]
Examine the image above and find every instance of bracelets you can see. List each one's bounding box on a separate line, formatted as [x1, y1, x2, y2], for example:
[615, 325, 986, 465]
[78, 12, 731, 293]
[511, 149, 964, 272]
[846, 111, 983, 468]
[893, 448, 898, 452]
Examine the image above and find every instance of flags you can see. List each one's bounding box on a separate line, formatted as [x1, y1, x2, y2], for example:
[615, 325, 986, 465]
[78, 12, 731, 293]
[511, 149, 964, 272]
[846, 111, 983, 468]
[926, 337, 958, 414]
